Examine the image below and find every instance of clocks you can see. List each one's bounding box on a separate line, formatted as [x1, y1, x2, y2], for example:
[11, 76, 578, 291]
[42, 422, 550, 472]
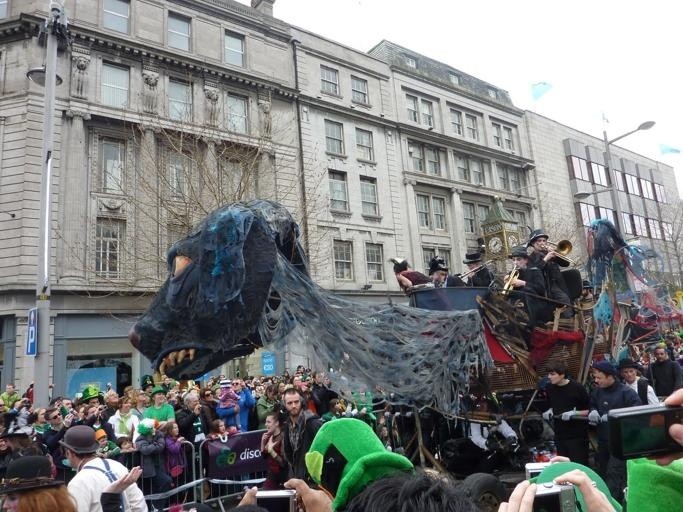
[507, 233, 518, 251]
[488, 236, 502, 254]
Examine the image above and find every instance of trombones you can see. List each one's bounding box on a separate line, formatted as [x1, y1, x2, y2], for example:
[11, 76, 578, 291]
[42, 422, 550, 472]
[543, 241, 581, 267]
[455, 259, 493, 280]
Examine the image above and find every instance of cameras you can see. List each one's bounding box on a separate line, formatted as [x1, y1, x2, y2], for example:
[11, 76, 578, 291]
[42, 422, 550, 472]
[255, 490, 297, 512]
[607, 404, 683, 461]
[525, 462, 550, 480]
[531, 481, 576, 512]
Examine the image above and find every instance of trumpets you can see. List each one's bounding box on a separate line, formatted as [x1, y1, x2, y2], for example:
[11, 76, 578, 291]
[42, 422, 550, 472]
[503, 266, 521, 296]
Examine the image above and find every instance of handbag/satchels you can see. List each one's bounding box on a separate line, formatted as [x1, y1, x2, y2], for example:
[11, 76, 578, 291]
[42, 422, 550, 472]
[171, 465, 183, 477]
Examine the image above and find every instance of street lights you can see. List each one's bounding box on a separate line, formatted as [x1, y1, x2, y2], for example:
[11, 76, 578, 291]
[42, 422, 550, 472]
[570, 119, 660, 304]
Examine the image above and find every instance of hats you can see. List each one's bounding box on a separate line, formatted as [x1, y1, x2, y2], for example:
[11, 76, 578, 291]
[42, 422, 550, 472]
[141, 375, 167, 398]
[463, 251, 482, 264]
[394, 265, 407, 272]
[0, 413, 34, 439]
[95, 429, 107, 440]
[583, 281, 594, 289]
[429, 256, 448, 275]
[220, 378, 232, 388]
[0, 456, 65, 496]
[80, 388, 103, 402]
[305, 418, 415, 512]
[508, 230, 548, 259]
[59, 425, 100, 454]
[592, 359, 640, 374]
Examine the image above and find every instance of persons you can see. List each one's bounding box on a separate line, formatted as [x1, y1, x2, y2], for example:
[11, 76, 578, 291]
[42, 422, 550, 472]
[391, 329, 683, 512]
[0, 366, 477, 512]
[387, 227, 595, 317]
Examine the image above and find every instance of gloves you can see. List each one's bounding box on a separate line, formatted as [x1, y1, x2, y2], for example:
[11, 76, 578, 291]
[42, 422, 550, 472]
[588, 410, 601, 426]
[601, 414, 608, 423]
[542, 408, 554, 421]
[559, 410, 576, 421]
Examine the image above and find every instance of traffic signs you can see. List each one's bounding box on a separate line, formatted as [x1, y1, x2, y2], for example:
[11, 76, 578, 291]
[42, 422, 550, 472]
[24, 308, 38, 357]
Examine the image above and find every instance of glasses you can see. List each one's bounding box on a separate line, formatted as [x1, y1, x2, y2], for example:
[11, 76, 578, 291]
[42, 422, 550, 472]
[232, 383, 238, 385]
[205, 393, 213, 397]
[46, 413, 61, 420]
